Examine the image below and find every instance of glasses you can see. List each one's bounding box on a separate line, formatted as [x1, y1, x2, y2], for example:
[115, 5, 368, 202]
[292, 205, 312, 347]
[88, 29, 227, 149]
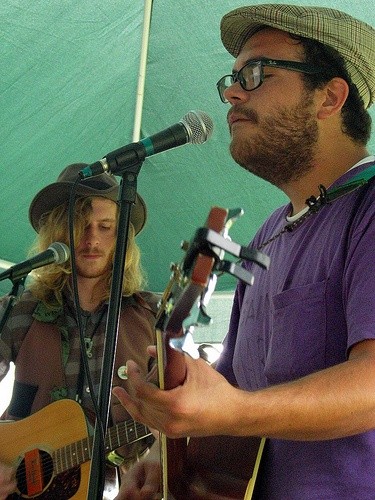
[216, 56, 341, 105]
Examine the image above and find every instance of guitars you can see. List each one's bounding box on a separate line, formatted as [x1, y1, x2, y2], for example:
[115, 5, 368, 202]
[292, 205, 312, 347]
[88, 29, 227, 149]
[140, 207, 267, 500]
[1, 398, 154, 500]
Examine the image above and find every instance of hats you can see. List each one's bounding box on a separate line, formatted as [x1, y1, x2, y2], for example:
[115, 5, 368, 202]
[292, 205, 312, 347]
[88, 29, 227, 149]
[220, 3, 375, 110]
[27, 160, 148, 239]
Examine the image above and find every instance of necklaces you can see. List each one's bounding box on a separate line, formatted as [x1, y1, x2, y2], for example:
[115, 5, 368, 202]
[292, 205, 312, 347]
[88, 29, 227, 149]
[72, 297, 106, 357]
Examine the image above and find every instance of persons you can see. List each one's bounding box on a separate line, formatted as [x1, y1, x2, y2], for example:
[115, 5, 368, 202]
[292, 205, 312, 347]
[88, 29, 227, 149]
[0, 162, 177, 500]
[100, 0, 374, 500]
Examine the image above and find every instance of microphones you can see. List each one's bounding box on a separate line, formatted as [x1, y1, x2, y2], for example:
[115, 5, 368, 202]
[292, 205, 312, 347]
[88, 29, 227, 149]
[1, 241, 71, 283]
[82, 110, 213, 183]
